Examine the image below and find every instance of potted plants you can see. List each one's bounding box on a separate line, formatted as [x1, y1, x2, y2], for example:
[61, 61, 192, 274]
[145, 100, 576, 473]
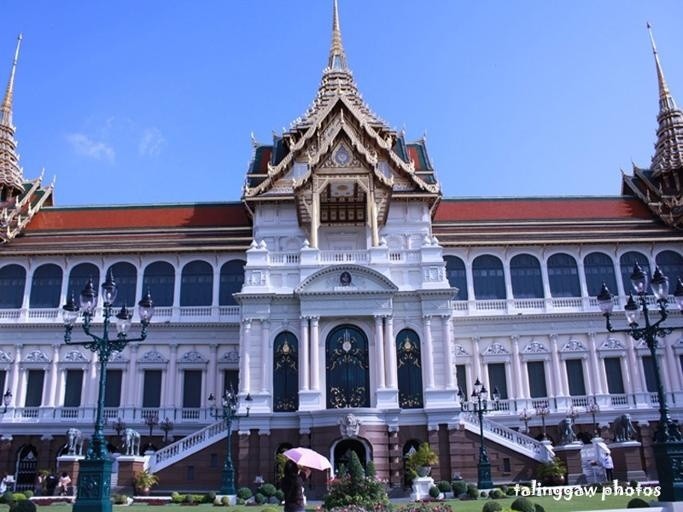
[136, 468, 159, 495]
[411, 442, 439, 477]
[542, 457, 567, 485]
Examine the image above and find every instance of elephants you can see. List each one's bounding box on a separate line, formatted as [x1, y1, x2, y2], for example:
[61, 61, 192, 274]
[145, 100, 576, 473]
[119, 428, 142, 456]
[63, 428, 83, 456]
[612, 413, 637, 442]
[558, 417, 576, 444]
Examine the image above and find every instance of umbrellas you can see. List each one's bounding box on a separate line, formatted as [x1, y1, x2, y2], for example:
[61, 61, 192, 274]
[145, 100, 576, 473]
[282, 446, 334, 472]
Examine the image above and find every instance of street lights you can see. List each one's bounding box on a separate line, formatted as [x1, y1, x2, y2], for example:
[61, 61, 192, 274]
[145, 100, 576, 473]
[49, 268, 153, 512]
[594, 259, 683, 503]
[455, 376, 501, 488]
[204, 383, 252, 492]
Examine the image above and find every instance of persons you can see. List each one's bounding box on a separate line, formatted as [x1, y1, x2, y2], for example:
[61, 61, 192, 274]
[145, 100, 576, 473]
[253, 471, 266, 490]
[55, 471, 72, 496]
[0, 471, 11, 494]
[44, 467, 61, 496]
[603, 451, 614, 482]
[279, 459, 312, 512]
[34, 472, 46, 496]
